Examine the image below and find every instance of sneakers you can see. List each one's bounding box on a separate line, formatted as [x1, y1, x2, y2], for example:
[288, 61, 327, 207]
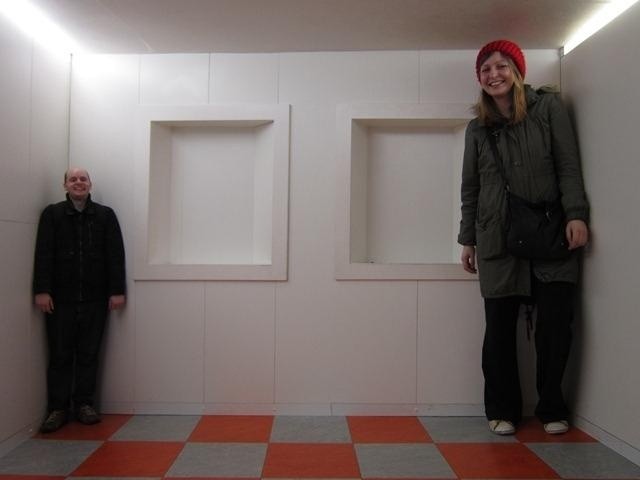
[543, 416, 569, 436]
[40, 403, 71, 434]
[76, 401, 101, 426]
[489, 417, 516, 436]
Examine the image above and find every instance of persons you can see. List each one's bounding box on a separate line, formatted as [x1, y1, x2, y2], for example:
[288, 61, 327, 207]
[32, 165, 127, 432]
[457, 39, 590, 437]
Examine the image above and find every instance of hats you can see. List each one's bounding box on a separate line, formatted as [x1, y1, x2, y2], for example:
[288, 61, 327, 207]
[475, 38, 526, 85]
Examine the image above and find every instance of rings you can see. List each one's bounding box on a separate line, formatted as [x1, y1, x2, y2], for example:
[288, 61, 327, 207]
[42, 306, 45, 309]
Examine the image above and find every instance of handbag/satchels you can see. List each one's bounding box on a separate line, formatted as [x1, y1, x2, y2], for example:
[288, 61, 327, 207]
[503, 191, 575, 262]
